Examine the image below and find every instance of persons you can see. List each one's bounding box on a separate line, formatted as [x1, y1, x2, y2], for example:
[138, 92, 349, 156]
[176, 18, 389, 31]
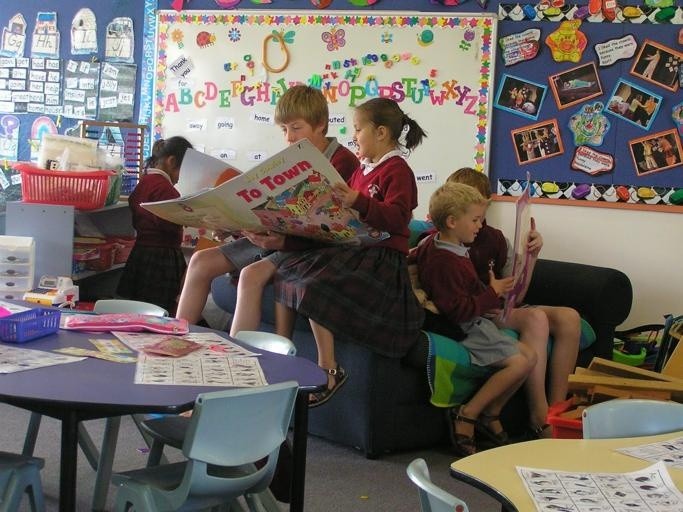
[171, 85, 360, 417]
[417, 181, 539, 455]
[267, 97, 429, 409]
[406, 166, 583, 440]
[117, 134, 212, 331]
[490, 46, 682, 181]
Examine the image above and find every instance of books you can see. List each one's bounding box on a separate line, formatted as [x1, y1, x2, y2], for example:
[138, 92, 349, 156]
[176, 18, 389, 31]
[135, 139, 390, 254]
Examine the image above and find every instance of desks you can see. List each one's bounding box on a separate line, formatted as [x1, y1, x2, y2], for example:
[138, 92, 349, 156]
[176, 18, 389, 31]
[448, 423, 683, 512]
[0, 297, 332, 512]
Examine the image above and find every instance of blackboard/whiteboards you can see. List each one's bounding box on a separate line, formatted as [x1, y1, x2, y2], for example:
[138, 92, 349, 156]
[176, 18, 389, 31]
[154, 8, 498, 231]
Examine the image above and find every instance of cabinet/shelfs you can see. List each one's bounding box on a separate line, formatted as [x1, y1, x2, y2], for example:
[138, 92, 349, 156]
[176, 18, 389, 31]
[4, 198, 143, 302]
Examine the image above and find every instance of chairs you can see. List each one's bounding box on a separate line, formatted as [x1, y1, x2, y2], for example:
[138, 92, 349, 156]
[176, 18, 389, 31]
[105, 378, 301, 512]
[405, 455, 470, 512]
[93, 298, 174, 485]
[582, 398, 681, 440]
[0, 449, 44, 512]
[139, 326, 298, 510]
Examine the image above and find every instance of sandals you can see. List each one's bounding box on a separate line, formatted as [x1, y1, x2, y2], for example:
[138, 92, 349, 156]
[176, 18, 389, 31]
[474, 410, 516, 446]
[446, 403, 477, 456]
[307, 360, 348, 408]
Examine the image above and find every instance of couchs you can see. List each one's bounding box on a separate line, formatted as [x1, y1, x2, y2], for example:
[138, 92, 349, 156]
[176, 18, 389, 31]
[209, 249, 633, 460]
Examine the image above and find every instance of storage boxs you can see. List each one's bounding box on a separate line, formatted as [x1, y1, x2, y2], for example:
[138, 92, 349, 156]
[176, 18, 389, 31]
[68, 235, 118, 270]
[545, 399, 584, 439]
[1, 234, 37, 296]
[109, 232, 139, 265]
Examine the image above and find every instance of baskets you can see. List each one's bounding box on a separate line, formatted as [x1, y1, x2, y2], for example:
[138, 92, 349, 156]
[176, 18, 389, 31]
[0, 307, 60, 343]
[11, 159, 122, 211]
